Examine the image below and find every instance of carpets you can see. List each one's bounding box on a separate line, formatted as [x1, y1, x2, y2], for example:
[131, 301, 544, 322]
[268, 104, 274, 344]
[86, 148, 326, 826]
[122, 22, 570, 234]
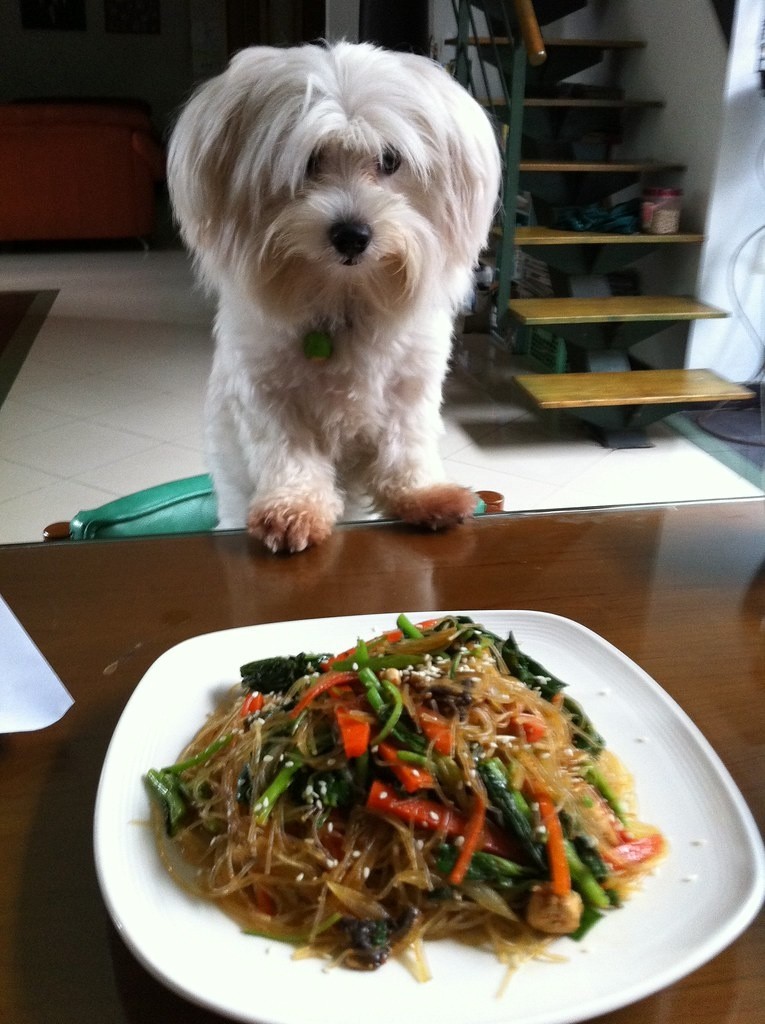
[0, 288, 62, 407]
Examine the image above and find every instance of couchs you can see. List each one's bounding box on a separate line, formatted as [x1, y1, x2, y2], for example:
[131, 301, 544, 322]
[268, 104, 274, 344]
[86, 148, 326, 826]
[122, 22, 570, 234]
[1, 97, 156, 249]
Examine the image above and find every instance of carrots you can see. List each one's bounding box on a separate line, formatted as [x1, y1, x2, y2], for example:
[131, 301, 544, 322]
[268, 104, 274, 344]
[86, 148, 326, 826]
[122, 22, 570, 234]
[242, 620, 663, 894]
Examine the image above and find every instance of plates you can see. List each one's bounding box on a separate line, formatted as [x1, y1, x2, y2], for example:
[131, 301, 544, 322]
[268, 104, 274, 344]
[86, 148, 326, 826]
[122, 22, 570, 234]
[94, 610, 765, 1024]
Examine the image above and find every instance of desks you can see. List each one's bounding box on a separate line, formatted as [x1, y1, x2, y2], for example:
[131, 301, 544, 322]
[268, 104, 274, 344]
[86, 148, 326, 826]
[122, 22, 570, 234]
[0, 495, 765, 1024]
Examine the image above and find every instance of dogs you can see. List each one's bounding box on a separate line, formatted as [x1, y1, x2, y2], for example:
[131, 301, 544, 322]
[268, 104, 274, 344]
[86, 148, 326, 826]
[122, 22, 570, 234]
[163, 33, 510, 555]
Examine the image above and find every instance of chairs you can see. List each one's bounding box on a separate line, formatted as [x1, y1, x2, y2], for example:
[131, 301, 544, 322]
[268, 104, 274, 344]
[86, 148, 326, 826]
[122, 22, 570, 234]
[42, 473, 504, 543]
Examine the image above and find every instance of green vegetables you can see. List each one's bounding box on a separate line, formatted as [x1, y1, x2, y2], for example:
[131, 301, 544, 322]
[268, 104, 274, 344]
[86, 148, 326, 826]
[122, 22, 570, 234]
[148, 614, 625, 946]
